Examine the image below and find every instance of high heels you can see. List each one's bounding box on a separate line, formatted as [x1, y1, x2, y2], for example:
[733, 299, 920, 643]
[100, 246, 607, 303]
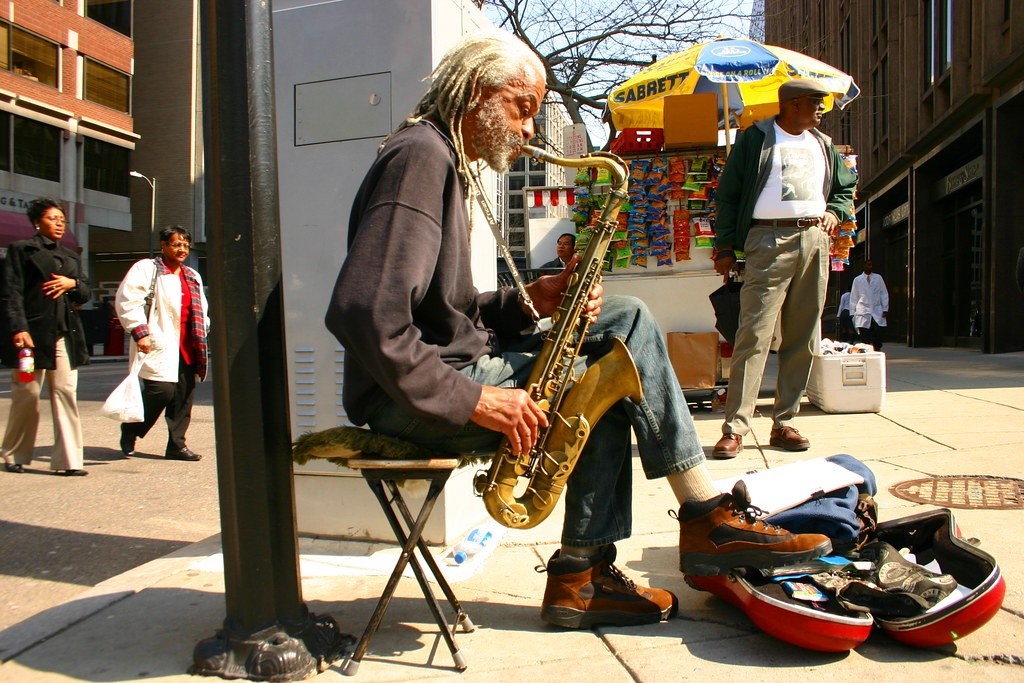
[5, 463, 24, 472]
[56, 470, 89, 476]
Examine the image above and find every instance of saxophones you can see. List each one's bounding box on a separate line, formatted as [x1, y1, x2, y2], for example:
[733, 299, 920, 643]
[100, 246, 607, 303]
[470, 142, 645, 532]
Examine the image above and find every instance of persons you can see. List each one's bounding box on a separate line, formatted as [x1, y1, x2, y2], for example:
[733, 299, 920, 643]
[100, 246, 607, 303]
[539, 233, 576, 275]
[712, 82, 857, 455]
[325, 30, 831, 629]
[836, 286, 854, 345]
[850, 261, 889, 351]
[116, 226, 210, 461]
[3, 196, 90, 476]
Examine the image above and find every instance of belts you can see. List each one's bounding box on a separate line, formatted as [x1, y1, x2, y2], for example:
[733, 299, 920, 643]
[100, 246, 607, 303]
[750, 218, 824, 227]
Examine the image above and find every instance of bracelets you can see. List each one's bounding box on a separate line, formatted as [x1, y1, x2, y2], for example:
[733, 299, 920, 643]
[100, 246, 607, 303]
[73, 276, 80, 288]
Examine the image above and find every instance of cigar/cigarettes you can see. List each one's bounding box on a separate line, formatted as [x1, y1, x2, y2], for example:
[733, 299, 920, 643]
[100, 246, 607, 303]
[52, 285, 56, 290]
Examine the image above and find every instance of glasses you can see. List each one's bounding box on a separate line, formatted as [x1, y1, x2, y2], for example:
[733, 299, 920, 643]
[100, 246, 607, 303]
[165, 241, 191, 249]
[794, 97, 824, 105]
[41, 215, 66, 223]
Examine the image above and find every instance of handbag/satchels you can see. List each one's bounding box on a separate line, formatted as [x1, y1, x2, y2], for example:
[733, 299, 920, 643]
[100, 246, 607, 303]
[99, 352, 149, 423]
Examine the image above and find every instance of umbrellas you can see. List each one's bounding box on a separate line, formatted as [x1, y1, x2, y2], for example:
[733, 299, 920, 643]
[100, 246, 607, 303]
[602, 34, 860, 157]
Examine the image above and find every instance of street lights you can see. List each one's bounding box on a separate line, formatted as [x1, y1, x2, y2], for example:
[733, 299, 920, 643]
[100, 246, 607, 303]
[129, 170, 156, 260]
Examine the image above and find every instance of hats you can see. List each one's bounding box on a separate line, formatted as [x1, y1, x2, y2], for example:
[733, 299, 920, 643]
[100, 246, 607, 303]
[778, 79, 829, 104]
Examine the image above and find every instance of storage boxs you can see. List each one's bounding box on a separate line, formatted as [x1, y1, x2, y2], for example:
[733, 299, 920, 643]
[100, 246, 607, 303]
[664, 92, 718, 149]
[731, 102, 779, 134]
[610, 128, 664, 156]
[666, 331, 722, 388]
[806, 352, 886, 413]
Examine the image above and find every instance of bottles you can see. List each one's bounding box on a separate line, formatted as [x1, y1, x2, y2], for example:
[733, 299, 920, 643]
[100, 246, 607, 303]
[19, 348, 34, 383]
[456, 523, 498, 563]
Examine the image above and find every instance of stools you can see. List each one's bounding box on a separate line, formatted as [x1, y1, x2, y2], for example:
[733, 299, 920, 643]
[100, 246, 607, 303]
[344, 459, 474, 675]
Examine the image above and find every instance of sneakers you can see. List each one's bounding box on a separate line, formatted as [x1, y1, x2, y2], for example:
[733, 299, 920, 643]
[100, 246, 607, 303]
[678, 480, 833, 575]
[540, 542, 679, 630]
[120, 433, 136, 455]
[166, 446, 202, 461]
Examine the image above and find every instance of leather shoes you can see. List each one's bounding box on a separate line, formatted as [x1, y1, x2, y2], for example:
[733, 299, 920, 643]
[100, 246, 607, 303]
[713, 433, 743, 458]
[769, 426, 810, 451]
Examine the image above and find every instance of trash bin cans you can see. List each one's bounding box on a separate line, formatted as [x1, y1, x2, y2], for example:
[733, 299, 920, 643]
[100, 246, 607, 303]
[497, 268, 566, 287]
[105, 318, 125, 356]
